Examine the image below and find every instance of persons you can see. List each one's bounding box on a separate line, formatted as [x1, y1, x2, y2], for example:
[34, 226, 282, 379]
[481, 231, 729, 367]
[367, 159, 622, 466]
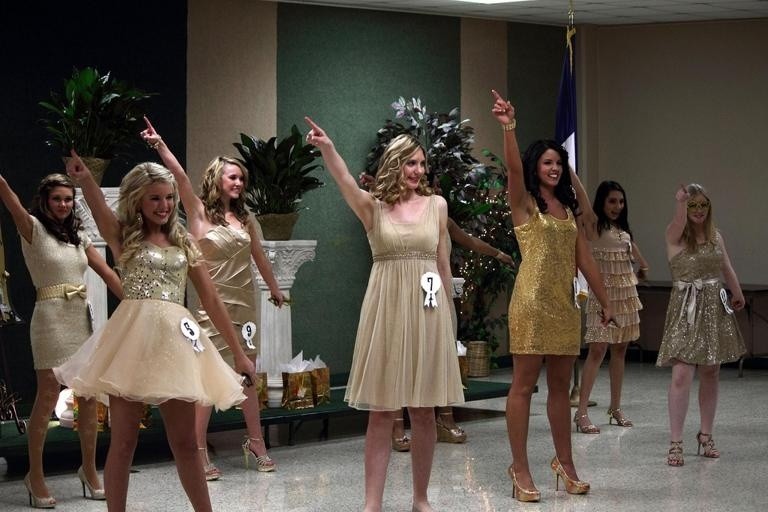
[1, 176, 126, 509]
[304, 115, 465, 512]
[139, 115, 285, 482]
[65, 148, 256, 512]
[655, 184, 745, 466]
[569, 165, 648, 435]
[489, 89, 591, 502]
[359, 172, 517, 451]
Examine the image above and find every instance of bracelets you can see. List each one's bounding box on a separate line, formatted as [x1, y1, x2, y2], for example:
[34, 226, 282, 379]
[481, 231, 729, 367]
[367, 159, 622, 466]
[495, 250, 504, 259]
[147, 136, 162, 148]
[639, 267, 650, 273]
[501, 118, 518, 131]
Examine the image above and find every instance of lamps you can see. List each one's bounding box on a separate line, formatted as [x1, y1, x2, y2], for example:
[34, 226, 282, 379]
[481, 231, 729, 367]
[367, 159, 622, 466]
[565, 0, 578, 77]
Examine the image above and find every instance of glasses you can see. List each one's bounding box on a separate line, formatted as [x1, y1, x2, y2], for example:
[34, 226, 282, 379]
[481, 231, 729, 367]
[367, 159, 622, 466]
[687, 199, 710, 212]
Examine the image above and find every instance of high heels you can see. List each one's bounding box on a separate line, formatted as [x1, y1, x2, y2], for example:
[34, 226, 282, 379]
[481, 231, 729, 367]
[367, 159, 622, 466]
[77, 466, 105, 499]
[574, 411, 599, 433]
[696, 430, 717, 457]
[550, 456, 590, 494]
[608, 407, 632, 426]
[569, 387, 596, 407]
[23, 470, 56, 507]
[242, 434, 276, 472]
[509, 463, 541, 502]
[199, 446, 221, 480]
[668, 439, 685, 467]
[435, 412, 467, 442]
[390, 418, 411, 452]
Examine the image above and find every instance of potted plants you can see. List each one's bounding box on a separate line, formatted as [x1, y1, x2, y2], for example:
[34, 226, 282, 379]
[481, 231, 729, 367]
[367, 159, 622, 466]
[228, 124, 326, 242]
[34, 61, 159, 187]
[447, 150, 519, 376]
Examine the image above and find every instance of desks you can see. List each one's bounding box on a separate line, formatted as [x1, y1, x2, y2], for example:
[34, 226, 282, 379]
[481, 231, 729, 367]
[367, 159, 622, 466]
[627, 280, 768, 378]
